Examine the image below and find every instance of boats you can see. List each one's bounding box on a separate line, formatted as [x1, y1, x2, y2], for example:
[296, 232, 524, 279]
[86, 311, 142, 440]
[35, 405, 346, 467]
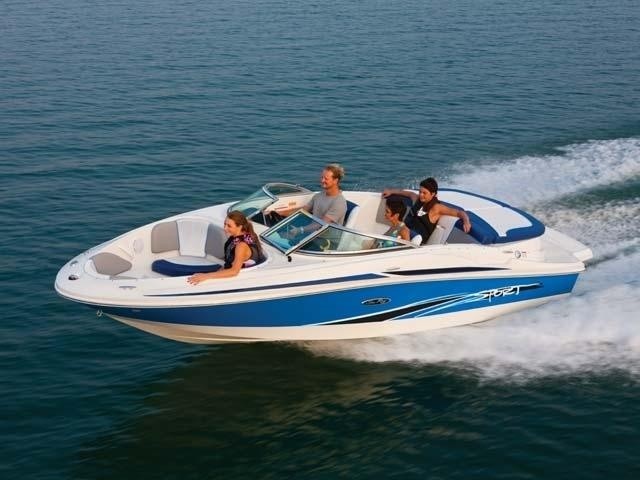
[55, 183, 594, 346]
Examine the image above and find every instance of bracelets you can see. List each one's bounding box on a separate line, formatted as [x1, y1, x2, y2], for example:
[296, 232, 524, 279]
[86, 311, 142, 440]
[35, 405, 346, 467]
[300, 227, 304, 234]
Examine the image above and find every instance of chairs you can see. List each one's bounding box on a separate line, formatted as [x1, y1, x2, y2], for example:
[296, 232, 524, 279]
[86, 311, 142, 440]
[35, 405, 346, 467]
[84, 218, 270, 279]
[331, 201, 498, 245]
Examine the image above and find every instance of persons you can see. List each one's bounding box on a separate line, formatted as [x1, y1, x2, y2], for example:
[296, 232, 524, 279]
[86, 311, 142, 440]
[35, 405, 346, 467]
[186, 211, 262, 285]
[265, 163, 348, 253]
[361, 193, 410, 253]
[382, 175, 471, 245]
